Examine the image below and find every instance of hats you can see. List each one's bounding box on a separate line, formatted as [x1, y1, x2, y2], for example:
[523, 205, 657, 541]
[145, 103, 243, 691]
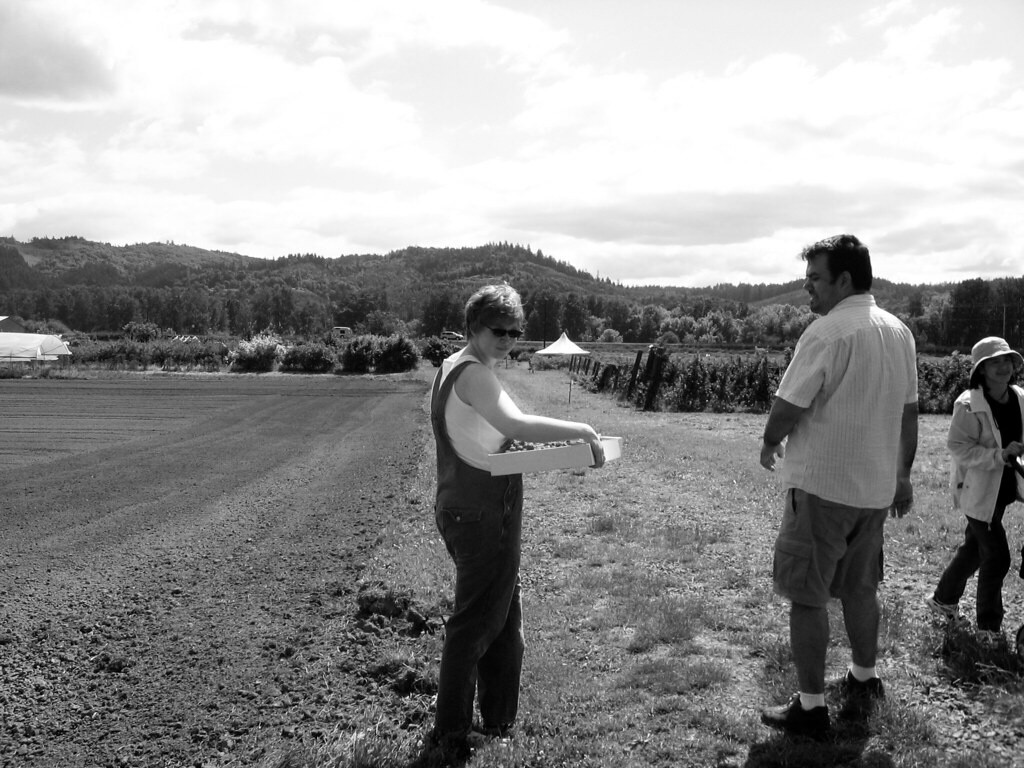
[970, 335, 1024, 387]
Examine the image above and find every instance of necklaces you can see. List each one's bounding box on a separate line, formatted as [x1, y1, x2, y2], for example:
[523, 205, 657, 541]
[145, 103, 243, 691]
[982, 387, 1009, 429]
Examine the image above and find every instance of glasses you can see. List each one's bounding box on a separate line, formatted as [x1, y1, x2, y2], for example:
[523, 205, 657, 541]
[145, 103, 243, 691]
[485, 321, 525, 339]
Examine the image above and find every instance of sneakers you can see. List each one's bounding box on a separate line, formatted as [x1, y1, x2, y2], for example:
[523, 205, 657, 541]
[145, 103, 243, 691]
[975, 631, 1013, 651]
[829, 670, 885, 702]
[926, 597, 970, 628]
[760, 692, 831, 732]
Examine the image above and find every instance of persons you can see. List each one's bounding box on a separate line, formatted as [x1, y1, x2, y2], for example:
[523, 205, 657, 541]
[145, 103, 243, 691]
[922, 335, 1024, 649]
[760, 234, 919, 734]
[415, 280, 606, 768]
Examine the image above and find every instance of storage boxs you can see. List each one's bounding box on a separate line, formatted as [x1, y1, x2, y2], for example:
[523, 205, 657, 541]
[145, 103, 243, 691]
[489, 435, 623, 477]
